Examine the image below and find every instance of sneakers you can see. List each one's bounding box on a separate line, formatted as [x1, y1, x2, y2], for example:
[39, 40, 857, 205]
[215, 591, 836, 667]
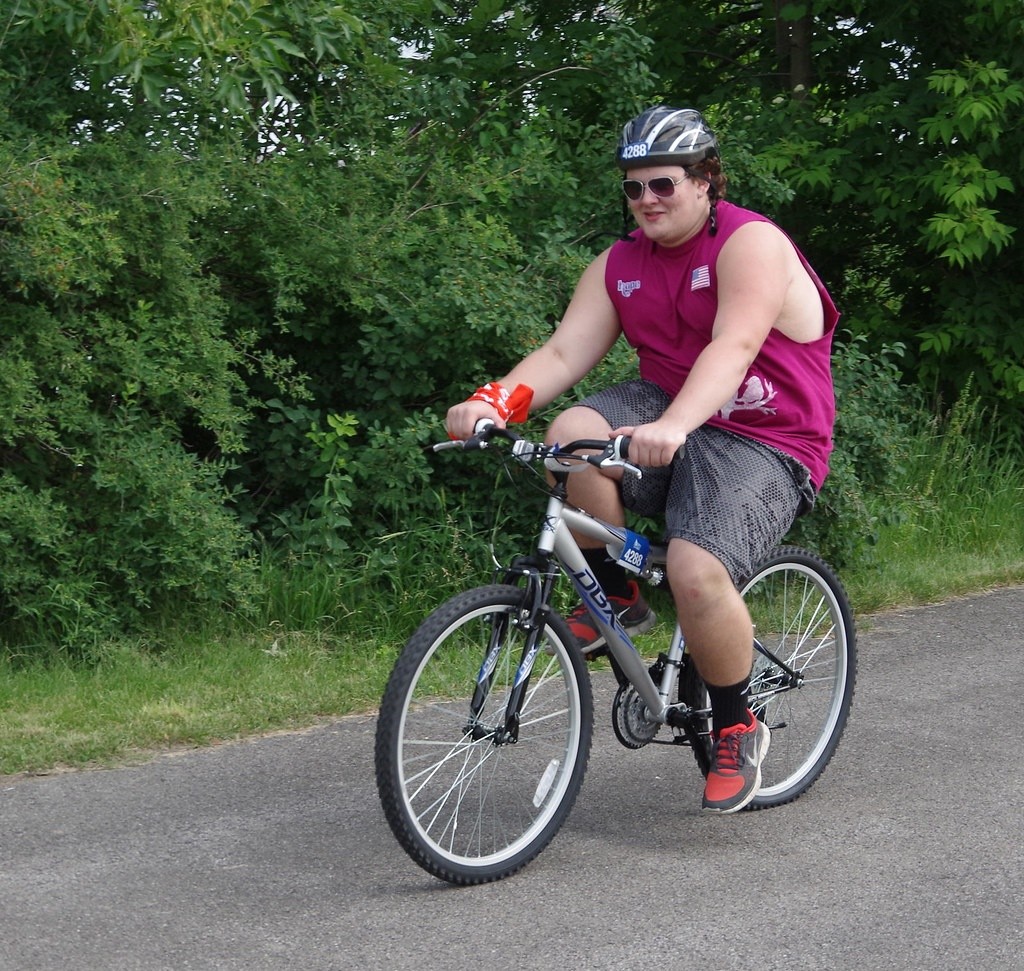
[702, 707, 771, 813]
[547, 579, 656, 654]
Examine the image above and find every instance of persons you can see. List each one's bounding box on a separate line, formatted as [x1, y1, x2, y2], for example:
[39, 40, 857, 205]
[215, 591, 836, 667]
[445, 107, 840, 814]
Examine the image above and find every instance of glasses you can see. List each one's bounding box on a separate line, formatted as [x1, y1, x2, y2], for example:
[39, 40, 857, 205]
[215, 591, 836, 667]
[622, 173, 691, 200]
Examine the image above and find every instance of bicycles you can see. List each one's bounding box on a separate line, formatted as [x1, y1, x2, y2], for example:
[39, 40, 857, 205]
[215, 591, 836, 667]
[373, 417, 857, 887]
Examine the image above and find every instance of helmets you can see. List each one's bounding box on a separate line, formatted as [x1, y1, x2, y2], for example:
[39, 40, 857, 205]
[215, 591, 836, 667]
[614, 105, 715, 170]
